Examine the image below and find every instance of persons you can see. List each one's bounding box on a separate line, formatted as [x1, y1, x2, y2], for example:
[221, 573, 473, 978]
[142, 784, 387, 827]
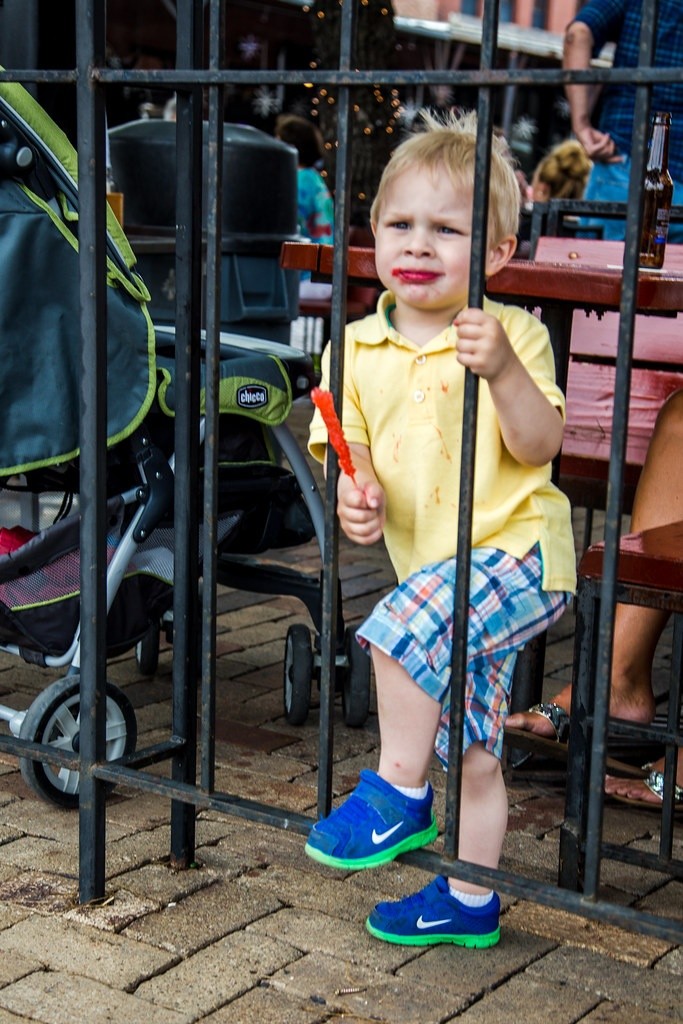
[305, 108, 577, 949]
[505, 390, 683, 808]
[276, 117, 334, 244]
[508, 0, 683, 242]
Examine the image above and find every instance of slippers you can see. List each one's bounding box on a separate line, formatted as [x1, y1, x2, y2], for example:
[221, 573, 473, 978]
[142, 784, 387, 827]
[504, 702, 648, 776]
[612, 763, 683, 809]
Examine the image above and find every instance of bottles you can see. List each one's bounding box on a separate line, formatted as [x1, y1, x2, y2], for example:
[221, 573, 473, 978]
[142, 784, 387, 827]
[639, 112, 674, 268]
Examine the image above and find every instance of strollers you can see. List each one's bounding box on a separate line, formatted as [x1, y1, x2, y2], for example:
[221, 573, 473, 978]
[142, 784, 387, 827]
[0, 64, 370, 812]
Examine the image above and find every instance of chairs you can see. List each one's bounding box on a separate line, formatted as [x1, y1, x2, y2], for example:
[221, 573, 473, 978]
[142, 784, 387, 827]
[297, 226, 380, 365]
[528, 238, 683, 558]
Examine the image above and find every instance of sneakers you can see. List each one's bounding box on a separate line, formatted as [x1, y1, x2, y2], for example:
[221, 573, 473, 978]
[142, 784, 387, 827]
[305, 769, 438, 870]
[366, 875, 500, 949]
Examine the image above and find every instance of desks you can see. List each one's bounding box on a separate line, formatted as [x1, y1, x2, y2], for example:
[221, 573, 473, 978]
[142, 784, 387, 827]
[278, 238, 683, 787]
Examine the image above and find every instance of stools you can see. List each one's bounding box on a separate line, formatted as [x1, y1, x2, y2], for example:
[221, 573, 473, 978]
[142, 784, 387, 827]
[558, 520, 682, 893]
